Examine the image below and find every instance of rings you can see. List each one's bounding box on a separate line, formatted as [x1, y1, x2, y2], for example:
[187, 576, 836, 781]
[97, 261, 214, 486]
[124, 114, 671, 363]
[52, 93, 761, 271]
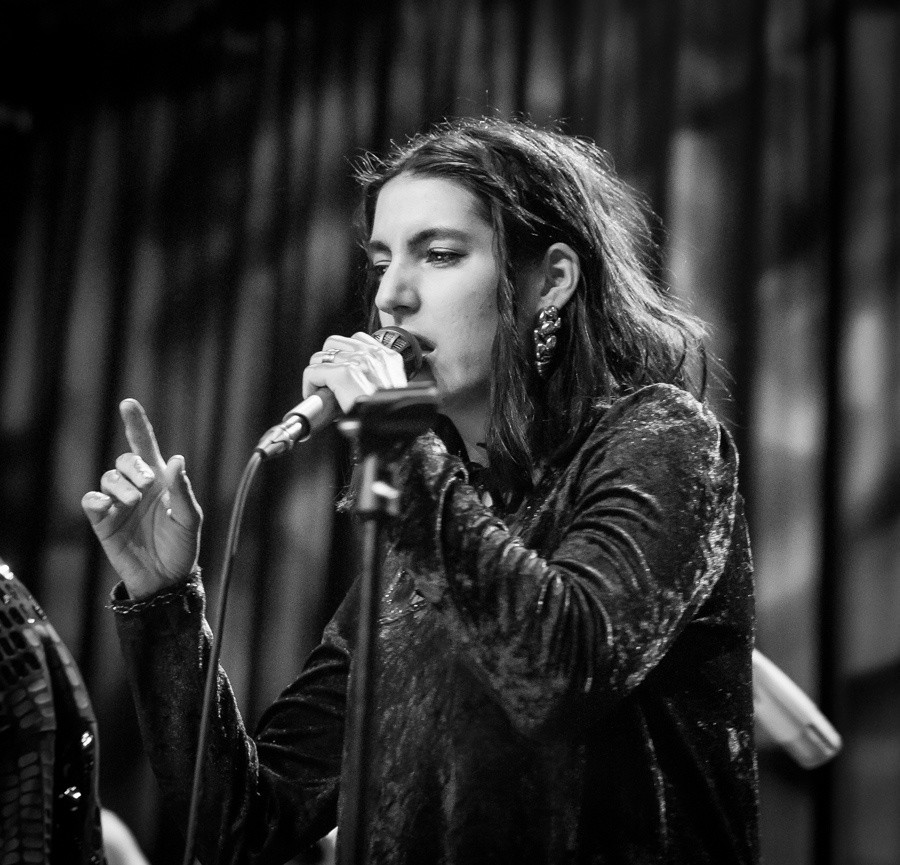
[321, 348, 340, 363]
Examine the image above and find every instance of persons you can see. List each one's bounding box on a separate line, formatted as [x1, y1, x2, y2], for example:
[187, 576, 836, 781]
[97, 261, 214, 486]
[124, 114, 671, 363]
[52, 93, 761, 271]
[81, 111, 761, 865]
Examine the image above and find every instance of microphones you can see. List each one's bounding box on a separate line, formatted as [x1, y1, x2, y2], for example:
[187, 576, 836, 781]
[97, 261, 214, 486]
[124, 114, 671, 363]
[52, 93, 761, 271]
[752, 648, 847, 773]
[253, 325, 423, 461]
